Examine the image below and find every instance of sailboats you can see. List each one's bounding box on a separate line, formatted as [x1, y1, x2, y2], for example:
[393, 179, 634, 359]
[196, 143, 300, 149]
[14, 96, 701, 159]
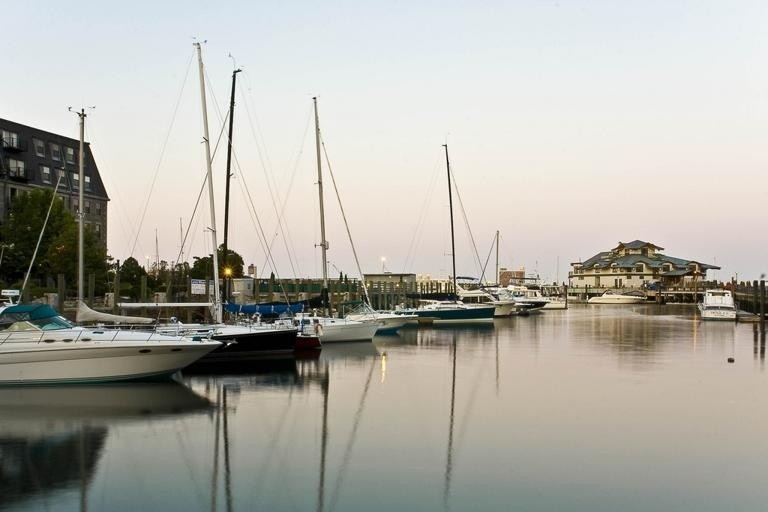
[386, 143, 498, 327]
[0, 105, 225, 384]
[293, 96, 385, 343]
[156, 41, 325, 371]
[0, 380, 237, 512]
[454, 231, 517, 318]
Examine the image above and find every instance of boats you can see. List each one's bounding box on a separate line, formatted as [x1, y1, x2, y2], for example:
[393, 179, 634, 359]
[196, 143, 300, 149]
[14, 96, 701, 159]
[584, 289, 648, 305]
[696, 287, 736, 321]
[344, 302, 423, 334]
[539, 285, 566, 309]
[497, 285, 551, 315]
